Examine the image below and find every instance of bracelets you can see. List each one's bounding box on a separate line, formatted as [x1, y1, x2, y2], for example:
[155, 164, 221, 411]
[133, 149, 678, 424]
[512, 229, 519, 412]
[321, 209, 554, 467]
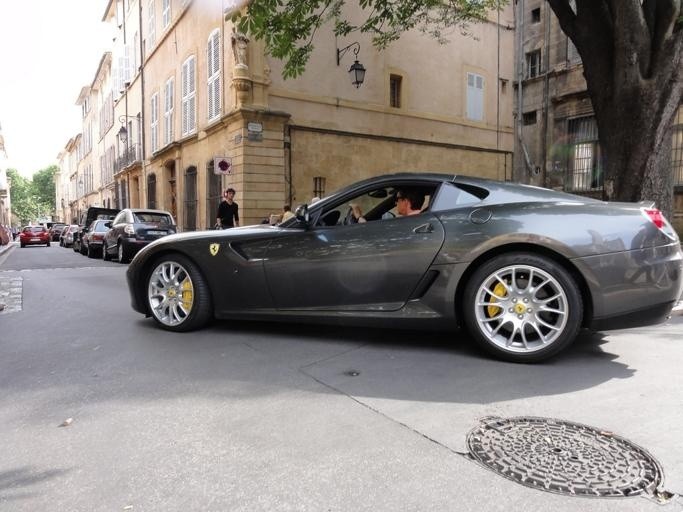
[356, 215, 362, 221]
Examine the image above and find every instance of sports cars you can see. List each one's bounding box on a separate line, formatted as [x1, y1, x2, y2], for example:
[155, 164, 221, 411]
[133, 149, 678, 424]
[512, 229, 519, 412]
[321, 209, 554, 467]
[124, 172, 683, 363]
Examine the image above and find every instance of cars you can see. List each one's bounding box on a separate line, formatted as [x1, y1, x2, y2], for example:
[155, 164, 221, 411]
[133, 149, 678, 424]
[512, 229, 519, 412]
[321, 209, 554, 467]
[12, 205, 178, 265]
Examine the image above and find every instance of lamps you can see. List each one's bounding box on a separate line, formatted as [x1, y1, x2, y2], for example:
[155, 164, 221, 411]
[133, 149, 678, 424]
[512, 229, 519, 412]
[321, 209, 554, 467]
[334, 41, 366, 92]
[76, 172, 89, 188]
[117, 114, 140, 144]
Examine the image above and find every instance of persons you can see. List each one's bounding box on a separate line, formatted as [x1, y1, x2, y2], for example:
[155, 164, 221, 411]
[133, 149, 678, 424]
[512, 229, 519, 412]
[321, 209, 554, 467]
[6, 226, 20, 243]
[346, 180, 425, 224]
[216, 188, 238, 230]
[260, 212, 275, 224]
[311, 197, 320, 203]
[280, 205, 294, 223]
